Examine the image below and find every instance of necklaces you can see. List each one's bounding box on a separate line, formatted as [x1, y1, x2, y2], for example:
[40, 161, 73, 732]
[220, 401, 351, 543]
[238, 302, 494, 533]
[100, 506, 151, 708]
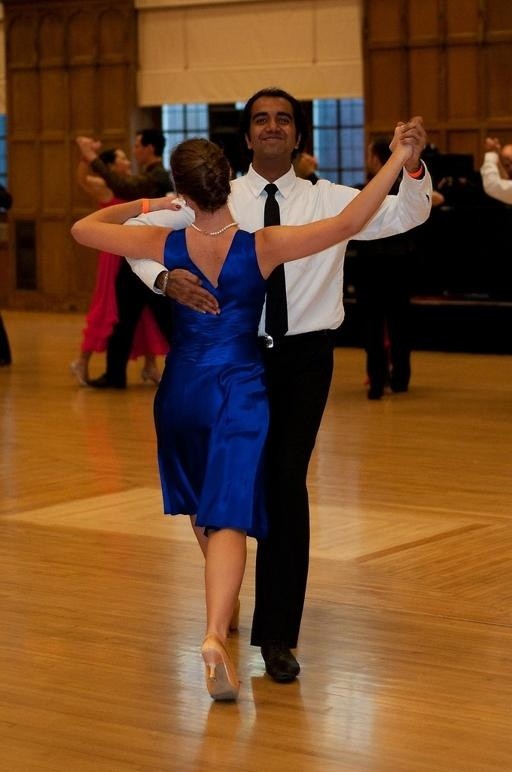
[191, 218, 237, 238]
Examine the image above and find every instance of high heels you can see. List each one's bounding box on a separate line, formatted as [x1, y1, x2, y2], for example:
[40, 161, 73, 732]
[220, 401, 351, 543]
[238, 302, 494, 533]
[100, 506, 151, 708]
[202, 635, 240, 700]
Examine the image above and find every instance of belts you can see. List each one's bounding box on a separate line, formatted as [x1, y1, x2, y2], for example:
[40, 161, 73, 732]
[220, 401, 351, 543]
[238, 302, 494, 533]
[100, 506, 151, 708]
[257, 331, 324, 348]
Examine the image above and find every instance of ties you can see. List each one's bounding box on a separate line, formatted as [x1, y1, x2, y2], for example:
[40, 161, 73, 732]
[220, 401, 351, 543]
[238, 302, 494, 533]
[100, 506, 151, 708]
[262, 184, 288, 344]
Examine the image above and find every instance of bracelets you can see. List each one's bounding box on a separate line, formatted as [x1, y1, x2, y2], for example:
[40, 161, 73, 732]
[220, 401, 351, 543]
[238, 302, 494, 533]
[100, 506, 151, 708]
[161, 272, 169, 295]
[408, 164, 422, 180]
[143, 197, 150, 214]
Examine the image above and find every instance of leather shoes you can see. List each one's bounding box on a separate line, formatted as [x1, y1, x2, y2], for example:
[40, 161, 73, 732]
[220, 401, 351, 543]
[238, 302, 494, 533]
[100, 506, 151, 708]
[142, 370, 162, 384]
[368, 374, 385, 399]
[69, 362, 87, 386]
[261, 647, 300, 682]
[89, 372, 116, 389]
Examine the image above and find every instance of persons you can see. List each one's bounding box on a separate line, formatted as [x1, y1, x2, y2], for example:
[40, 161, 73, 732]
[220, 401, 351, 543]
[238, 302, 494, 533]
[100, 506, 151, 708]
[478, 132, 511, 205]
[75, 128, 179, 388]
[121, 89, 431, 683]
[1, 179, 11, 369]
[68, 121, 426, 702]
[73, 133, 160, 386]
[360, 145, 442, 392]
[303, 135, 418, 399]
